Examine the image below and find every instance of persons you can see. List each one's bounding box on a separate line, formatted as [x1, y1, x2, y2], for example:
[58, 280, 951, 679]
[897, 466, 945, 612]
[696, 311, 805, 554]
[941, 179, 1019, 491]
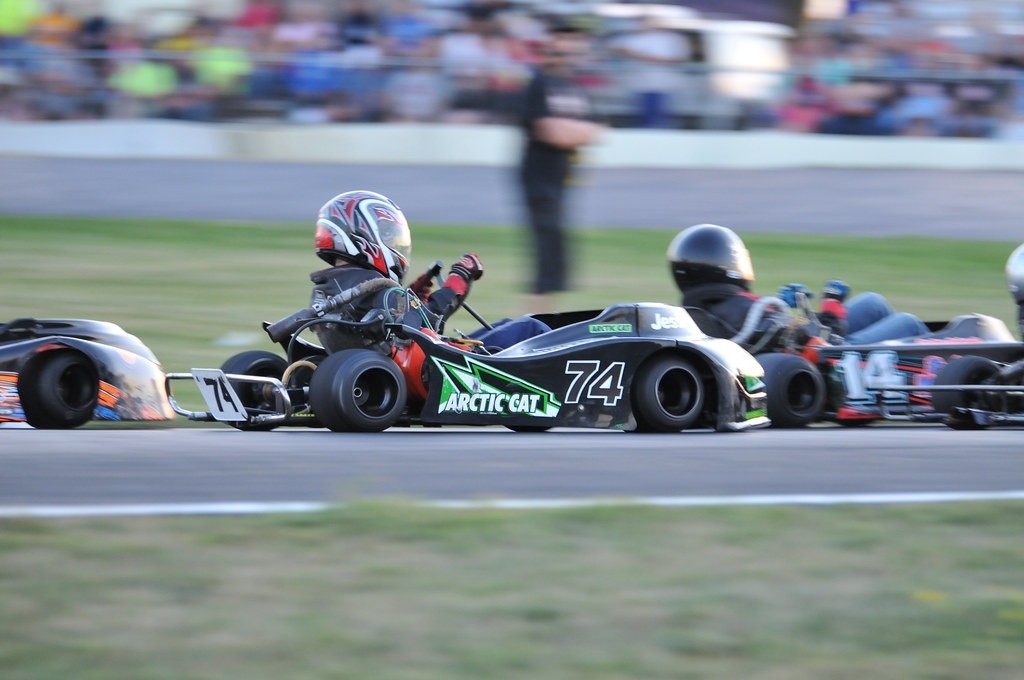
[309, 190, 552, 352]
[665, 225, 932, 349]
[520, 32, 608, 293]
[0, 0, 1020, 139]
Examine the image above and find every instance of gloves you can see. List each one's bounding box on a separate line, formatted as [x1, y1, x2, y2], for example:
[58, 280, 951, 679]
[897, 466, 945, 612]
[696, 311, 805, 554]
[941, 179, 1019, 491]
[823, 280, 849, 303]
[778, 283, 812, 306]
[406, 275, 432, 304]
[450, 252, 482, 283]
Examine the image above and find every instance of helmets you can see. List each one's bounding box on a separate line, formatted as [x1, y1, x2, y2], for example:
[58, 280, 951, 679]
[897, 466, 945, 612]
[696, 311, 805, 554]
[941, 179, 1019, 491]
[315, 189, 411, 284]
[669, 223, 754, 292]
[1006, 242, 1024, 305]
